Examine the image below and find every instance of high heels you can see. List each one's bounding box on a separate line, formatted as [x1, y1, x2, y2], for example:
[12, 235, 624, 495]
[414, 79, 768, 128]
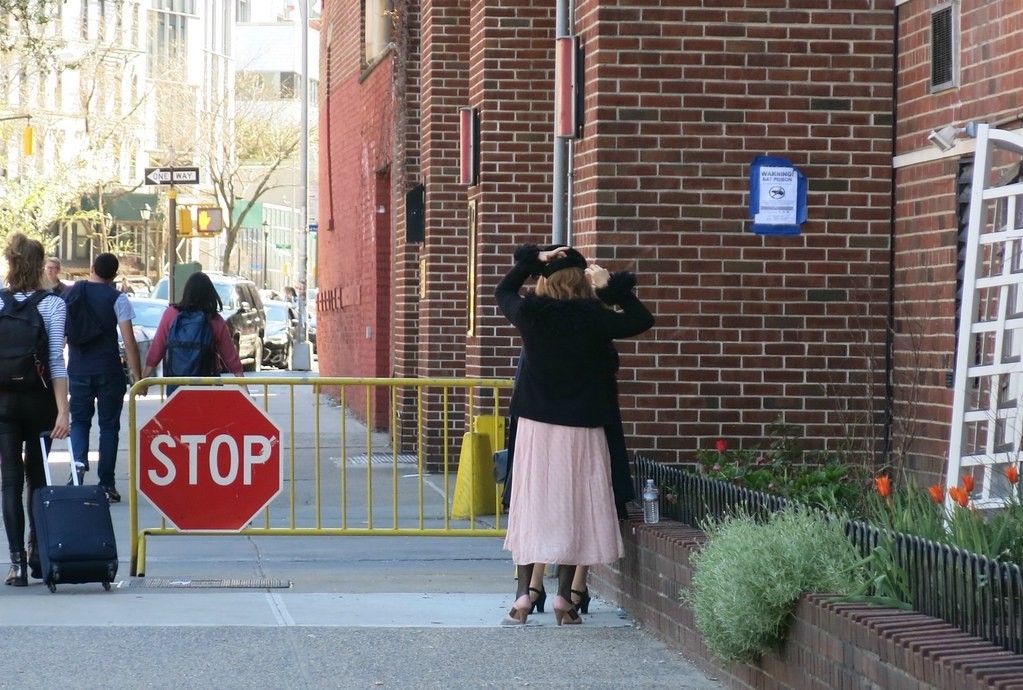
[553, 596, 582, 626]
[571, 586, 591, 614]
[508, 594, 532, 624]
[529, 585, 547, 613]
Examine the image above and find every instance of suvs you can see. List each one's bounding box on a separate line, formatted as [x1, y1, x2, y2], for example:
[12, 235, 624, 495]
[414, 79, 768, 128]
[152, 272, 267, 374]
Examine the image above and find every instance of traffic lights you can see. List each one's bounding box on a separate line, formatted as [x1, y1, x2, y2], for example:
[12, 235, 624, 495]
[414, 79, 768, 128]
[197, 208, 222, 232]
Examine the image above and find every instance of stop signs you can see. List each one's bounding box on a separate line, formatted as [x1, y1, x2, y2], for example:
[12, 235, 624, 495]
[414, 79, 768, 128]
[136, 385, 285, 533]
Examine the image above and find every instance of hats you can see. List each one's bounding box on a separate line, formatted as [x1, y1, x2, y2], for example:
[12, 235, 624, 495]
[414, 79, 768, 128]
[542, 244, 587, 279]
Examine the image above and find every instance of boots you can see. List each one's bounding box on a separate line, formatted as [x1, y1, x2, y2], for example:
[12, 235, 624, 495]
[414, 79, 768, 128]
[28, 531, 42, 578]
[4, 551, 27, 586]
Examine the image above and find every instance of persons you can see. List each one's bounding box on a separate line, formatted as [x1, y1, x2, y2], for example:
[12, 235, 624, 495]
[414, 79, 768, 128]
[0, 233, 70, 587]
[120, 278, 135, 297]
[59, 253, 142, 502]
[495, 242, 655, 624]
[43, 257, 68, 348]
[143, 274, 250, 398]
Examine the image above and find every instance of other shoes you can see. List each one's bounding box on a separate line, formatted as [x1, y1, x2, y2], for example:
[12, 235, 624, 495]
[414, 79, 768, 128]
[100, 485, 121, 502]
[66, 462, 85, 486]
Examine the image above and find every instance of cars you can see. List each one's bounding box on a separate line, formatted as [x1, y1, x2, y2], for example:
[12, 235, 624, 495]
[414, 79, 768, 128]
[260, 299, 299, 370]
[113, 274, 154, 295]
[260, 288, 318, 355]
[62, 296, 174, 386]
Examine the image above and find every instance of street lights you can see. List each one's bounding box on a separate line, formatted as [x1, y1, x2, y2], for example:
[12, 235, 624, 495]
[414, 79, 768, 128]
[140, 201, 152, 277]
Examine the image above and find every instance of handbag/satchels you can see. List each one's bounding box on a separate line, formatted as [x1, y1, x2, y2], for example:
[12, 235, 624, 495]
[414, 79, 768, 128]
[493, 449, 509, 483]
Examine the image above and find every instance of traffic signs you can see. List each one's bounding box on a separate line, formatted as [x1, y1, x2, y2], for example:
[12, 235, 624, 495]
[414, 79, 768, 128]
[144, 167, 199, 186]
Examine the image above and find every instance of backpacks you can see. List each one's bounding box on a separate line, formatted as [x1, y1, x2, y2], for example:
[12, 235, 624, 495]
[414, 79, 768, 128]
[165, 308, 213, 376]
[0, 288, 57, 386]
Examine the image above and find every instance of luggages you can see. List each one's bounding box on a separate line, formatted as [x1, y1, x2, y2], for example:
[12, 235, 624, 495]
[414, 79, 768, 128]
[32, 431, 118, 592]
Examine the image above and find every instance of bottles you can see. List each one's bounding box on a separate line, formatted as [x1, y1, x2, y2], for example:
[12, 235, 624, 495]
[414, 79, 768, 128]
[643, 479, 660, 524]
